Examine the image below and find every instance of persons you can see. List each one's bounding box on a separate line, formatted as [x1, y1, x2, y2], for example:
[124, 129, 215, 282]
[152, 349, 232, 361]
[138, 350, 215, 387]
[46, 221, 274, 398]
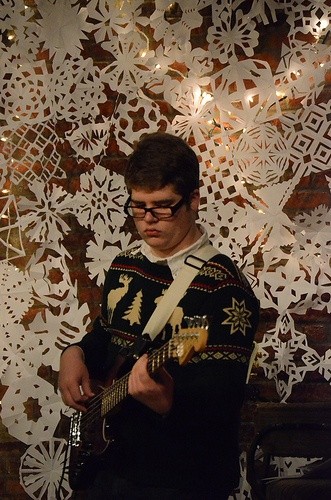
[60, 133, 257, 500]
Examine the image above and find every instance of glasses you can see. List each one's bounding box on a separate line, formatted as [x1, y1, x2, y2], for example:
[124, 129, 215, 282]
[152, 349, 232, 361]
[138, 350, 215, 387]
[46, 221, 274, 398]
[124, 192, 193, 219]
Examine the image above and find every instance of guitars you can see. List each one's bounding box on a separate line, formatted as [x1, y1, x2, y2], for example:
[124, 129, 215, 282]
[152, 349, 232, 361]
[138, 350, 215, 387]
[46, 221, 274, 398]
[70, 314, 210, 489]
